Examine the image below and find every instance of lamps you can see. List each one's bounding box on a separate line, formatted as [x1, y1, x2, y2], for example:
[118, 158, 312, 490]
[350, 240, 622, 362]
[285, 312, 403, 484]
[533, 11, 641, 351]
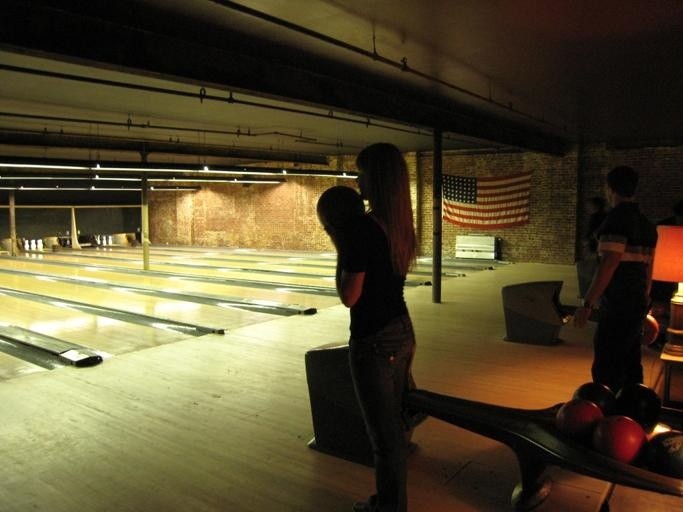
[651, 225, 682, 358]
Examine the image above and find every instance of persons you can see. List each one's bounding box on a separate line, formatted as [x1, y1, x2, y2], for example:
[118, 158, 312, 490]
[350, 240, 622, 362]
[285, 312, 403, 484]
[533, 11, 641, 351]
[572, 166, 659, 392]
[317, 143, 422, 511]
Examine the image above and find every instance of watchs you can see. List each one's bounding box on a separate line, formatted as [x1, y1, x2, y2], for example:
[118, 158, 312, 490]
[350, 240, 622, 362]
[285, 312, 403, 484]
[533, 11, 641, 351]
[579, 298, 593, 309]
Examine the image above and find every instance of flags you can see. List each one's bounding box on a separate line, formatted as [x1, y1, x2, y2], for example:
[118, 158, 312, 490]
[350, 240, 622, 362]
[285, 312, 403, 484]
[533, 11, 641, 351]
[442, 170, 535, 230]
